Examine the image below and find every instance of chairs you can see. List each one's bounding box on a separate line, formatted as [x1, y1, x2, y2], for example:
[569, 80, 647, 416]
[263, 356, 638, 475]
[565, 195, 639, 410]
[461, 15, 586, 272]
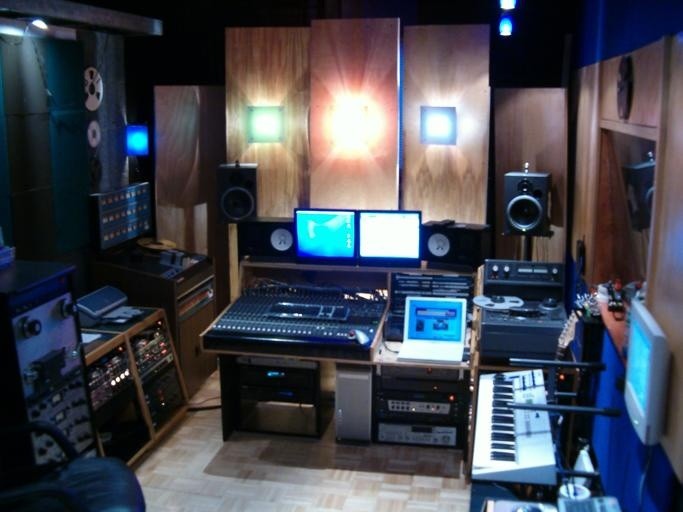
[0, 420, 146, 512]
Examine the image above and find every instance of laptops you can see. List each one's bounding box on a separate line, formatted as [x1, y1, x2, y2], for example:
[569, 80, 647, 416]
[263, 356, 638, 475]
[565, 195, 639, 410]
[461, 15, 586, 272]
[396, 295, 468, 366]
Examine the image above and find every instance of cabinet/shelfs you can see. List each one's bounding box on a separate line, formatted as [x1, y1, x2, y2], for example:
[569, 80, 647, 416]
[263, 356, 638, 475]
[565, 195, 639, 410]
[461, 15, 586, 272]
[78, 306, 190, 467]
[110, 262, 218, 400]
[197, 260, 482, 463]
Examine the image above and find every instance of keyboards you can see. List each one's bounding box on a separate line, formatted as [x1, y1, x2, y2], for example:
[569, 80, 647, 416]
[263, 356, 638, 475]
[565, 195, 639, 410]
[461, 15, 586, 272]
[267, 301, 351, 321]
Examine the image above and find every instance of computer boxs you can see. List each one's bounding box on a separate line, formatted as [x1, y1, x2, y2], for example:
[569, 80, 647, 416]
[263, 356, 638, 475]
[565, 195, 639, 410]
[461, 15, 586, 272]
[333, 363, 376, 444]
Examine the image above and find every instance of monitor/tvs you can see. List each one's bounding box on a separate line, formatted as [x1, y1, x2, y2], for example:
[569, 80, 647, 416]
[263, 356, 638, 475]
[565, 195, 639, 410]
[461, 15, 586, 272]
[358, 210, 422, 268]
[623, 297, 671, 446]
[294, 207, 358, 266]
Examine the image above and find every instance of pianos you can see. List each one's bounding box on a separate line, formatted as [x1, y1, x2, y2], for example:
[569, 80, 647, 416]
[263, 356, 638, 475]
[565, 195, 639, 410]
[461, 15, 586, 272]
[471, 369, 558, 485]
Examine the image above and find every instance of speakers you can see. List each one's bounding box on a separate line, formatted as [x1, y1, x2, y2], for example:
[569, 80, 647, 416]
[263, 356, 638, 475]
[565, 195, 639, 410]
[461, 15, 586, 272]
[422, 220, 492, 274]
[621, 159, 656, 233]
[503, 171, 552, 236]
[237, 216, 296, 263]
[218, 163, 260, 224]
